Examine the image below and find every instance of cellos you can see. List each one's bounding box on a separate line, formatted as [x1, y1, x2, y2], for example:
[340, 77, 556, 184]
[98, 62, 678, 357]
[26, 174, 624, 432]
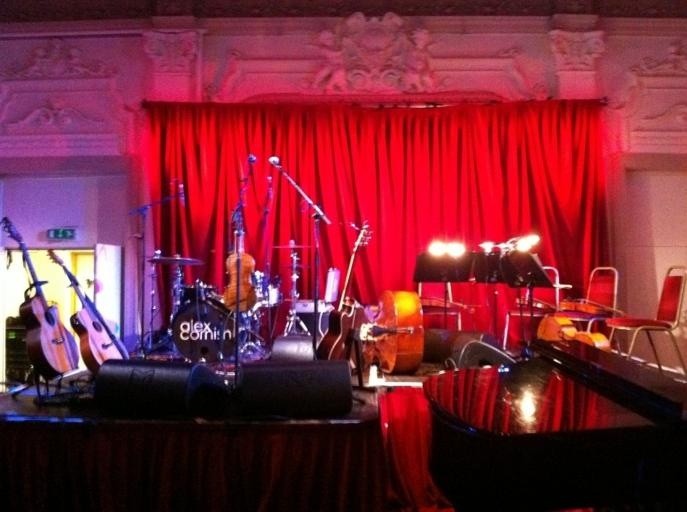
[1, 217, 78, 378]
[47, 249, 131, 374]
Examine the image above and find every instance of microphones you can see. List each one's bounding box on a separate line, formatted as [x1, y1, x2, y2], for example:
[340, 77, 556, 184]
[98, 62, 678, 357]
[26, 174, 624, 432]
[179, 183, 185, 207]
[268, 156, 279, 165]
[248, 153, 256, 164]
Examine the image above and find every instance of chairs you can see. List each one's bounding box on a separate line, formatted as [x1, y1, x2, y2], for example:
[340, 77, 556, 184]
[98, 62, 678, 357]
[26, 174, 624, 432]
[605, 265, 687, 376]
[502, 266, 559, 350]
[554, 266, 622, 357]
[418, 281, 463, 331]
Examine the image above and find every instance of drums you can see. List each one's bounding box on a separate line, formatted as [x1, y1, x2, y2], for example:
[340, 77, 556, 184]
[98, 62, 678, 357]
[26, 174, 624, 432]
[290, 300, 326, 314]
[169, 295, 237, 362]
[172, 285, 214, 307]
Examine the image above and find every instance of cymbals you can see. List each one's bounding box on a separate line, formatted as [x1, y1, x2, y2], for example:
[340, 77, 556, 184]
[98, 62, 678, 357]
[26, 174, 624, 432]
[270, 245, 317, 248]
[147, 257, 204, 267]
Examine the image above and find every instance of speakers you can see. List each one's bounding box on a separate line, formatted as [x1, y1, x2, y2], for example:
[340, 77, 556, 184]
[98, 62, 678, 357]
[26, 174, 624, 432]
[449, 332, 517, 382]
[240, 361, 352, 421]
[95, 358, 230, 418]
[271, 336, 326, 361]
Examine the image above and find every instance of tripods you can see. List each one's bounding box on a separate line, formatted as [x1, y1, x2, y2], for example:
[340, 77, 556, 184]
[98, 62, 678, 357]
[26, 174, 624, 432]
[235, 314, 266, 359]
[284, 253, 312, 336]
[120, 195, 174, 359]
[511, 287, 548, 370]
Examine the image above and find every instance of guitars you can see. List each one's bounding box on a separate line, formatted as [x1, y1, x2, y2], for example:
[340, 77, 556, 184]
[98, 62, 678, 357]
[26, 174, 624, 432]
[318, 222, 370, 365]
[360, 290, 425, 375]
[223, 210, 257, 311]
